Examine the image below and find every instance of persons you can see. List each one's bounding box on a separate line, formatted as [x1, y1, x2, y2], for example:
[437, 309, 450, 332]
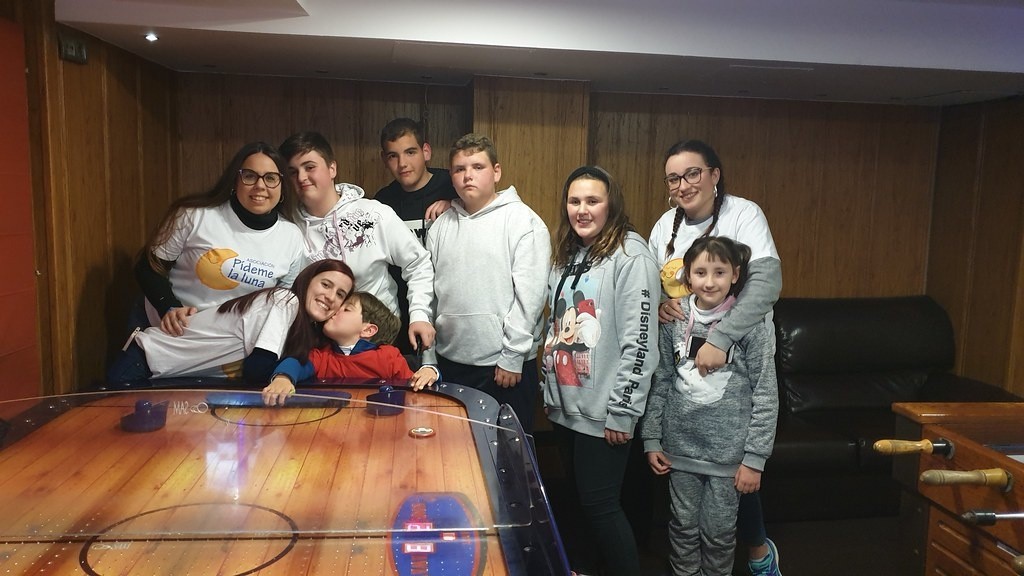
[278, 130, 436, 351]
[421, 133, 553, 446]
[106, 259, 356, 386]
[372, 118, 461, 380]
[538, 166, 673, 576]
[648, 138, 783, 576]
[640, 236, 780, 576]
[129, 141, 303, 337]
[261, 291, 442, 407]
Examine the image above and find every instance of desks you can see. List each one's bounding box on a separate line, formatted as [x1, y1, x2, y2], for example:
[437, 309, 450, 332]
[0, 377, 571, 576]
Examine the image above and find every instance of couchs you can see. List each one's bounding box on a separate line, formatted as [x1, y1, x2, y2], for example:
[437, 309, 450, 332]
[619, 295, 1024, 525]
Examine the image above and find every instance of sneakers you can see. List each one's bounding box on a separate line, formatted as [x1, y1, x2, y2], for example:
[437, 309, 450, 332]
[747, 537, 782, 576]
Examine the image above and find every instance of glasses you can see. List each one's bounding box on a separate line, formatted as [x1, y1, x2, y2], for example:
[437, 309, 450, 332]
[663, 167, 713, 191]
[235, 169, 283, 188]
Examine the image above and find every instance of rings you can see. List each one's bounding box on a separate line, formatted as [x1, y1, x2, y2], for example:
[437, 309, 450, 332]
[708, 366, 718, 373]
[663, 304, 668, 311]
